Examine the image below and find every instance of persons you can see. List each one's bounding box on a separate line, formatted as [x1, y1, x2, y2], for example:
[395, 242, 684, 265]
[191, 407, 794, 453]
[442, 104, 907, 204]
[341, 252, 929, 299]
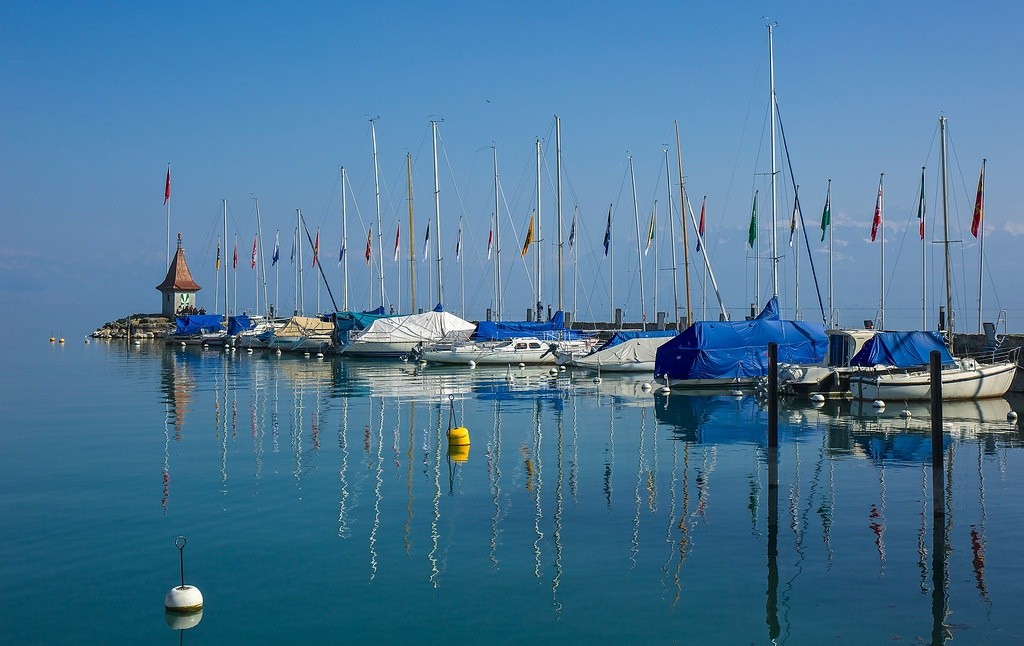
[269, 304, 274, 316]
[177, 305, 206, 314]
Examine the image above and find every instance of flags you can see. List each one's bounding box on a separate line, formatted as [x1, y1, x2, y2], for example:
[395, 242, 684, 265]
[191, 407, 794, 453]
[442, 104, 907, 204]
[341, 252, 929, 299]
[251, 240, 257, 269]
[455, 222, 462, 262]
[338, 237, 344, 265]
[216, 241, 220, 270]
[870, 182, 883, 242]
[819, 189, 830, 242]
[788, 192, 798, 247]
[971, 171, 982, 238]
[644, 211, 654, 254]
[917, 174, 925, 238]
[747, 196, 756, 248]
[272, 234, 279, 266]
[395, 226, 400, 261]
[568, 213, 576, 247]
[696, 203, 704, 251]
[164, 168, 170, 204]
[521, 215, 534, 257]
[291, 235, 296, 264]
[234, 243, 237, 267]
[604, 211, 610, 256]
[488, 221, 493, 259]
[422, 223, 429, 262]
[365, 231, 371, 262]
[313, 233, 318, 267]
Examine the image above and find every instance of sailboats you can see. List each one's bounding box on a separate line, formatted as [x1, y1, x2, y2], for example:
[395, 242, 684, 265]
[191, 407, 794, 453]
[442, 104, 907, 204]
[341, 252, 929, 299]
[166, 112, 477, 355]
[654, 15, 839, 389]
[783, 394, 1024, 646]
[571, 372, 673, 568]
[849, 111, 1020, 401]
[787, 166, 947, 400]
[400, 136, 600, 365]
[571, 120, 708, 372]
[657, 382, 848, 646]
